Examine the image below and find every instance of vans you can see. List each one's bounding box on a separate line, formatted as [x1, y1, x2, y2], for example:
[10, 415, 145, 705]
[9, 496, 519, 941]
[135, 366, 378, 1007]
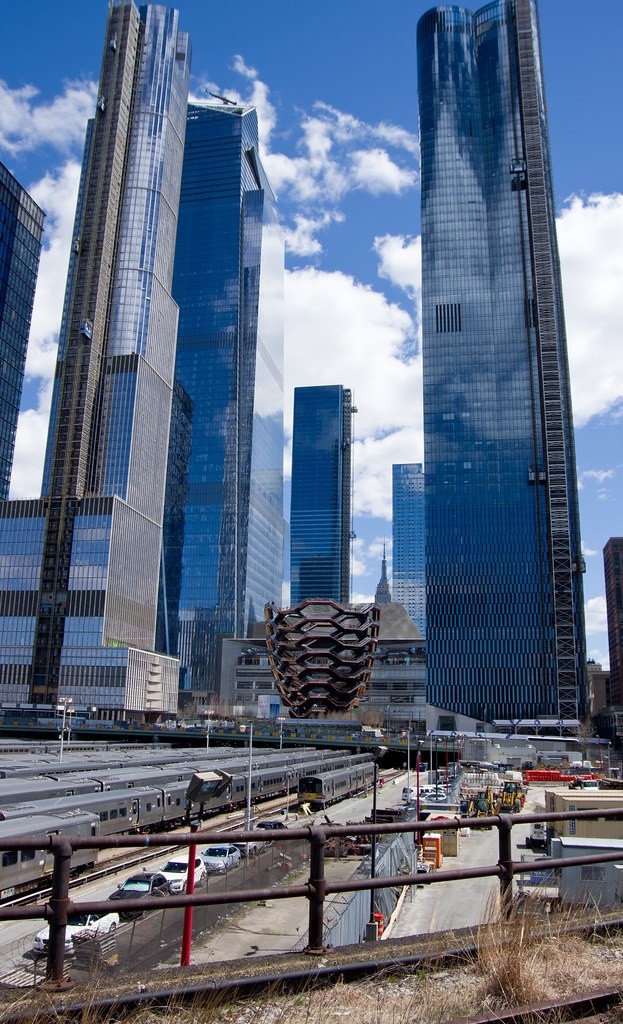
[401, 787, 439, 801]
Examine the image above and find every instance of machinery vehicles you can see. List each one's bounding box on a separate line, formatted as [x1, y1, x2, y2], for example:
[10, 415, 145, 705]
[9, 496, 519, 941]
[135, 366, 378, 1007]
[459, 785, 500, 830]
[496, 779, 522, 813]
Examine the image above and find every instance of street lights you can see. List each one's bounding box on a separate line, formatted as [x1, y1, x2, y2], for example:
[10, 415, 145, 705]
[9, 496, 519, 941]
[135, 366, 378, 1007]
[59, 698, 74, 763]
[240, 724, 252, 831]
[178, 768, 233, 965]
[368, 735, 458, 924]
[278, 717, 285, 749]
[204, 710, 214, 748]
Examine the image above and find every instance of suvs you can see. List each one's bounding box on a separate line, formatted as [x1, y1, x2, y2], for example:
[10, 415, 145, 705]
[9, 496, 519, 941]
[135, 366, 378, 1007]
[256, 821, 288, 830]
[156, 855, 208, 891]
[106, 873, 171, 921]
[411, 793, 447, 803]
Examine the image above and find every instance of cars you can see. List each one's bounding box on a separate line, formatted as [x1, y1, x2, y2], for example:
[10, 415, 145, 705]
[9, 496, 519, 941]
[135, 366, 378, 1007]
[32, 910, 119, 954]
[199, 845, 241, 875]
[229, 841, 266, 858]
[432, 787, 447, 793]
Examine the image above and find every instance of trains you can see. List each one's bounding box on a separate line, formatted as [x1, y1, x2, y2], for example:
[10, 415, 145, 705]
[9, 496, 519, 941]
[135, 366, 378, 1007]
[0, 739, 375, 835]
[297, 762, 379, 810]
[0, 807, 100, 909]
